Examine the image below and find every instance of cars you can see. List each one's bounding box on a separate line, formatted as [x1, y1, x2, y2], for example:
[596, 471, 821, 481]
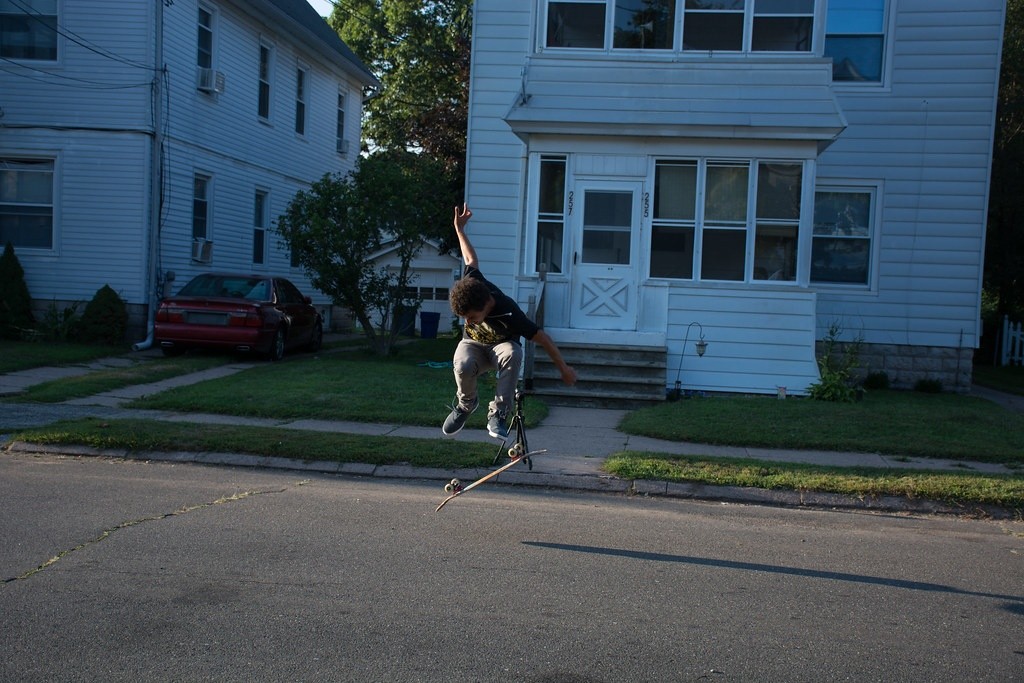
[153, 272, 327, 362]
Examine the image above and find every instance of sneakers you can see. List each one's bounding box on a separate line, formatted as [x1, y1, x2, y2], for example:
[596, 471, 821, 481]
[486, 412, 509, 442]
[441, 396, 480, 436]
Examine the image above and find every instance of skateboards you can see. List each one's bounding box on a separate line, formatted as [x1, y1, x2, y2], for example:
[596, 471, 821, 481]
[434, 443, 547, 512]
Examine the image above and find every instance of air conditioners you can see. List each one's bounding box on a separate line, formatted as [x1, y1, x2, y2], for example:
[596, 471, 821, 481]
[337, 140, 349, 153]
[192, 240, 213, 264]
[198, 67, 225, 96]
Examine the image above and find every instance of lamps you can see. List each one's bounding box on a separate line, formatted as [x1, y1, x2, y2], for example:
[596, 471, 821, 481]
[668, 322, 709, 400]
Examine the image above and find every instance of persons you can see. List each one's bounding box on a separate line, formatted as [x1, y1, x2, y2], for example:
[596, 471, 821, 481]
[442, 203, 578, 441]
